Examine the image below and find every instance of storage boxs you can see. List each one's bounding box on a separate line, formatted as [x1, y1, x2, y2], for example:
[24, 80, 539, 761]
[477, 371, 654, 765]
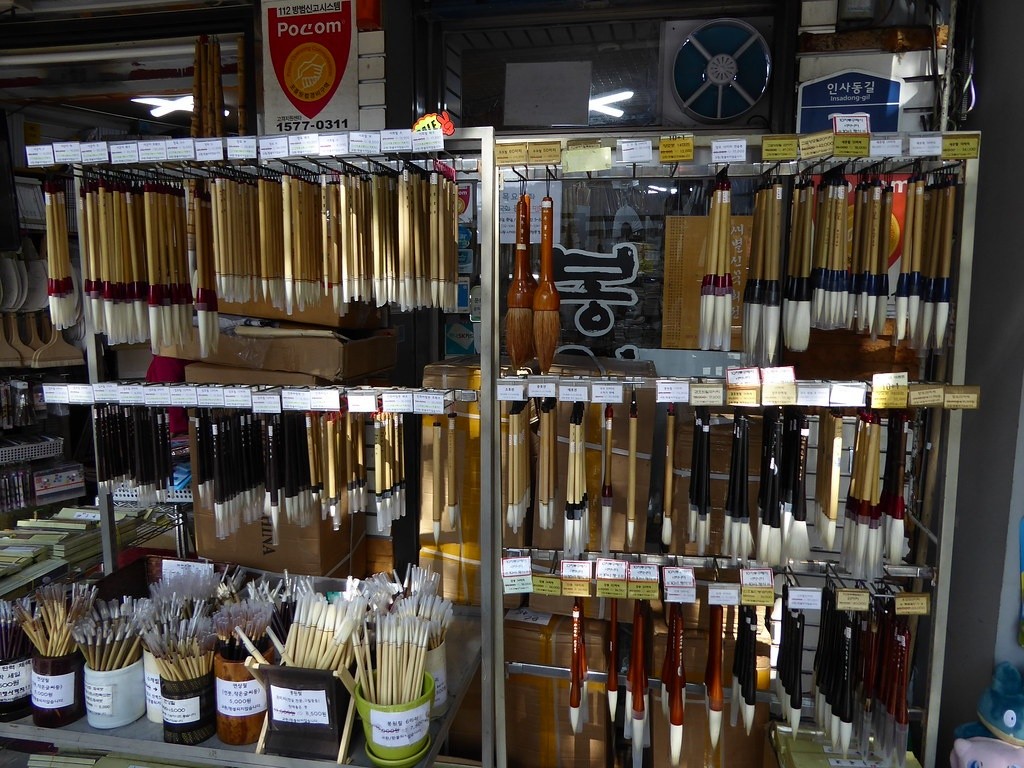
[33, 460, 85, 496]
[421, 356, 771, 768]
[110, 339, 153, 379]
[153, 185, 397, 579]
[766, 719, 923, 768]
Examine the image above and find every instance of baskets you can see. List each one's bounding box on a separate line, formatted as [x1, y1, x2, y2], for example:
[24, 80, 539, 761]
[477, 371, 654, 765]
[0, 437, 64, 466]
[112, 482, 194, 502]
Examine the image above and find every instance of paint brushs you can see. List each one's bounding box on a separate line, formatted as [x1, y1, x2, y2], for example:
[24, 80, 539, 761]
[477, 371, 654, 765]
[507, 198, 558, 374]
[93, 406, 911, 583]
[46, 169, 459, 357]
[568, 605, 911, 762]
[699, 173, 955, 362]
[0, 564, 455, 706]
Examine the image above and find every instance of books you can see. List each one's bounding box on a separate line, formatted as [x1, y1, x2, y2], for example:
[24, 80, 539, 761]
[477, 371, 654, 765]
[771, 721, 922, 768]
[0, 506, 168, 605]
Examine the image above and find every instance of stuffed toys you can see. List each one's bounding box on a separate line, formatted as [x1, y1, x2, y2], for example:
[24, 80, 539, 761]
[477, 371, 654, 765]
[954, 661, 1024, 747]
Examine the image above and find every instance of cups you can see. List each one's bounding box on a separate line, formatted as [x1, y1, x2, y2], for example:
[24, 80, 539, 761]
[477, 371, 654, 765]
[213, 643, 274, 746]
[404, 639, 449, 719]
[83, 660, 146, 729]
[0, 651, 40, 722]
[142, 645, 164, 724]
[30, 649, 84, 728]
[356, 664, 436, 768]
